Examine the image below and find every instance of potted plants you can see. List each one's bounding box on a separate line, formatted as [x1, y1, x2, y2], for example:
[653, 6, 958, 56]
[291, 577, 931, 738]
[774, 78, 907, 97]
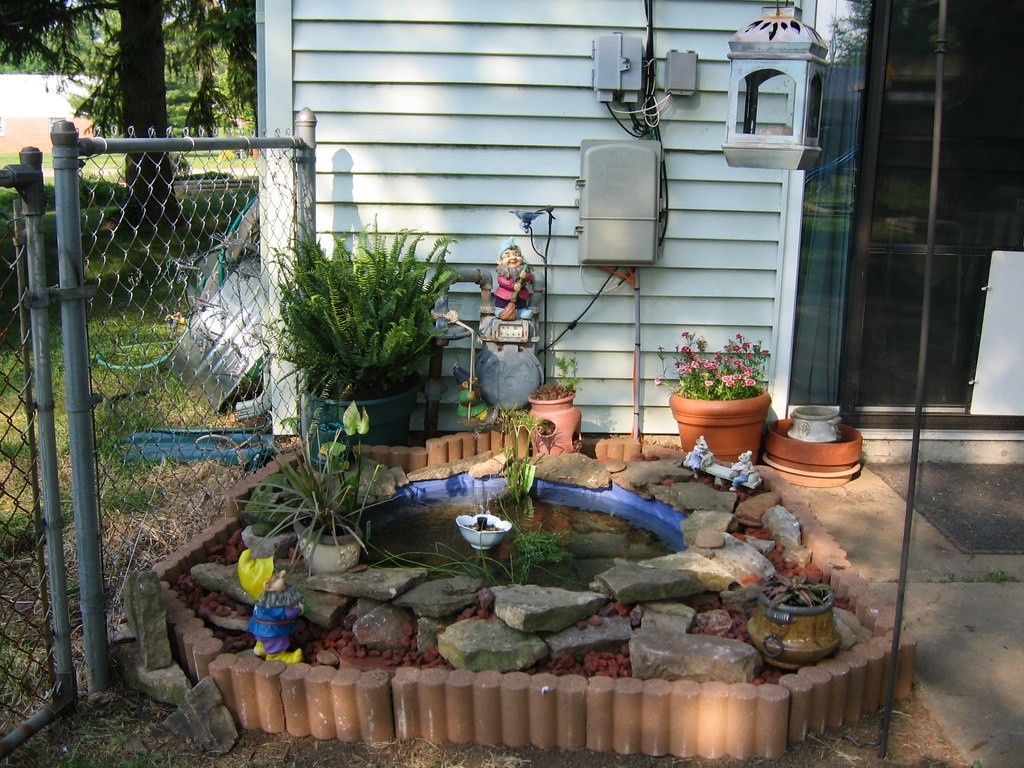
[748, 572, 841, 671]
[261, 212, 464, 447]
[230, 400, 385, 576]
[526, 350, 582, 455]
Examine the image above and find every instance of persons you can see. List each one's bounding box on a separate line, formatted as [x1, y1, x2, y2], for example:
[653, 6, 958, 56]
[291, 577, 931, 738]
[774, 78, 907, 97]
[494, 236, 534, 319]
[453, 365, 488, 422]
[237, 549, 310, 664]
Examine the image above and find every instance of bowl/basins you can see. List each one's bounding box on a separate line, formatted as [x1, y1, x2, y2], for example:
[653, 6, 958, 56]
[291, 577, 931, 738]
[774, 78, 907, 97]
[455, 513, 512, 550]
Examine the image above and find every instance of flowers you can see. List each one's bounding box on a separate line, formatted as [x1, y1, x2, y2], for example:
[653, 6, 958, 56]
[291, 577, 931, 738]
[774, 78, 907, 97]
[654, 332, 770, 401]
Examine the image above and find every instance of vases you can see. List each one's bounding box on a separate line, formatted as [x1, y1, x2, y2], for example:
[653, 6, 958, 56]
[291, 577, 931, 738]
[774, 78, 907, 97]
[669, 390, 772, 466]
[764, 419, 863, 473]
[787, 407, 844, 443]
